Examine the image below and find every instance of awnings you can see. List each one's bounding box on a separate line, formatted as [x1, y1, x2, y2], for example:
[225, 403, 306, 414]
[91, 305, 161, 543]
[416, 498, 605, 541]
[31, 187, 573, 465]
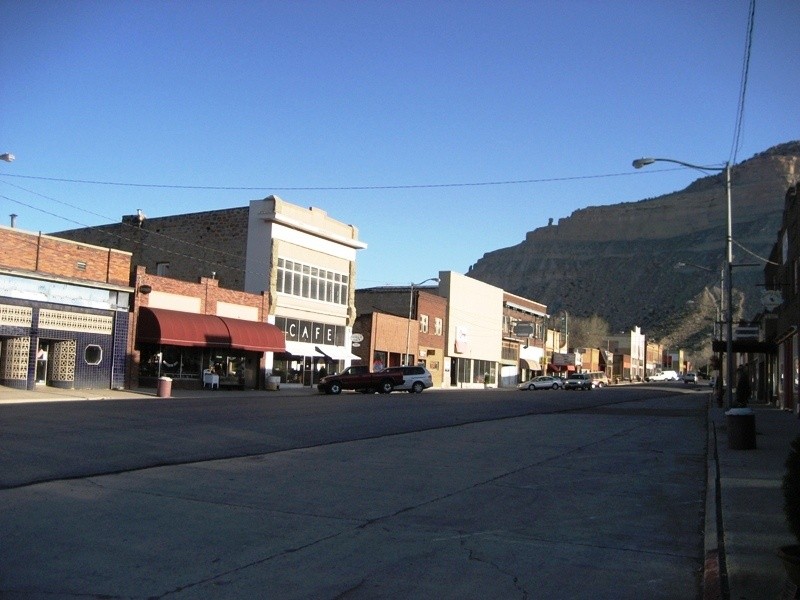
[562, 365, 576, 371]
[285, 344, 325, 357]
[315, 345, 363, 360]
[547, 364, 560, 373]
[136, 306, 285, 353]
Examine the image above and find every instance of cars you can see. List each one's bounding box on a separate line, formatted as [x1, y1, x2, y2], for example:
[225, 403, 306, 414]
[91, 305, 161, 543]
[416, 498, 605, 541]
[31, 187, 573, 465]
[518, 375, 563, 391]
[547, 371, 609, 390]
[565, 373, 593, 391]
[684, 373, 699, 385]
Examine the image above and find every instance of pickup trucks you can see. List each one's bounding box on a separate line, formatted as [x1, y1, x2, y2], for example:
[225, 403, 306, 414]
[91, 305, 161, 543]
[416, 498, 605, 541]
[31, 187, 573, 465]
[317, 364, 405, 395]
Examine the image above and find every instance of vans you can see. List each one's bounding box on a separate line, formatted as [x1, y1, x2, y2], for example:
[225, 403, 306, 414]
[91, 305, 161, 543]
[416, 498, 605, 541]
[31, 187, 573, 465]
[377, 365, 433, 394]
[649, 370, 684, 382]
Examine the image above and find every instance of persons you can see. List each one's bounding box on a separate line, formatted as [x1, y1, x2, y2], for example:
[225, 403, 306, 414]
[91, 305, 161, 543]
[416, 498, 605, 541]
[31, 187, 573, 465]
[484, 371, 490, 389]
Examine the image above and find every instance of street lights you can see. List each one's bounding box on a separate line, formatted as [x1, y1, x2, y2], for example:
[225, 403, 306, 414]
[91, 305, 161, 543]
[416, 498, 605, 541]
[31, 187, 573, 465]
[632, 156, 733, 412]
[405, 277, 441, 367]
[677, 260, 725, 408]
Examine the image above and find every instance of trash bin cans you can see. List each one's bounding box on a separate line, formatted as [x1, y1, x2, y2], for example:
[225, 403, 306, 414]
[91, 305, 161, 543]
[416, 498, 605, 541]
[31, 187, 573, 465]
[157, 376, 172, 398]
[725, 408, 758, 450]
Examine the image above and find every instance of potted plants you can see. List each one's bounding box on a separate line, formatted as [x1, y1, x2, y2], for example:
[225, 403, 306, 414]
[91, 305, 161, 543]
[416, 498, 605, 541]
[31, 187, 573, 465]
[774, 431, 800, 600]
[726, 373, 756, 450]
[715, 380, 725, 407]
